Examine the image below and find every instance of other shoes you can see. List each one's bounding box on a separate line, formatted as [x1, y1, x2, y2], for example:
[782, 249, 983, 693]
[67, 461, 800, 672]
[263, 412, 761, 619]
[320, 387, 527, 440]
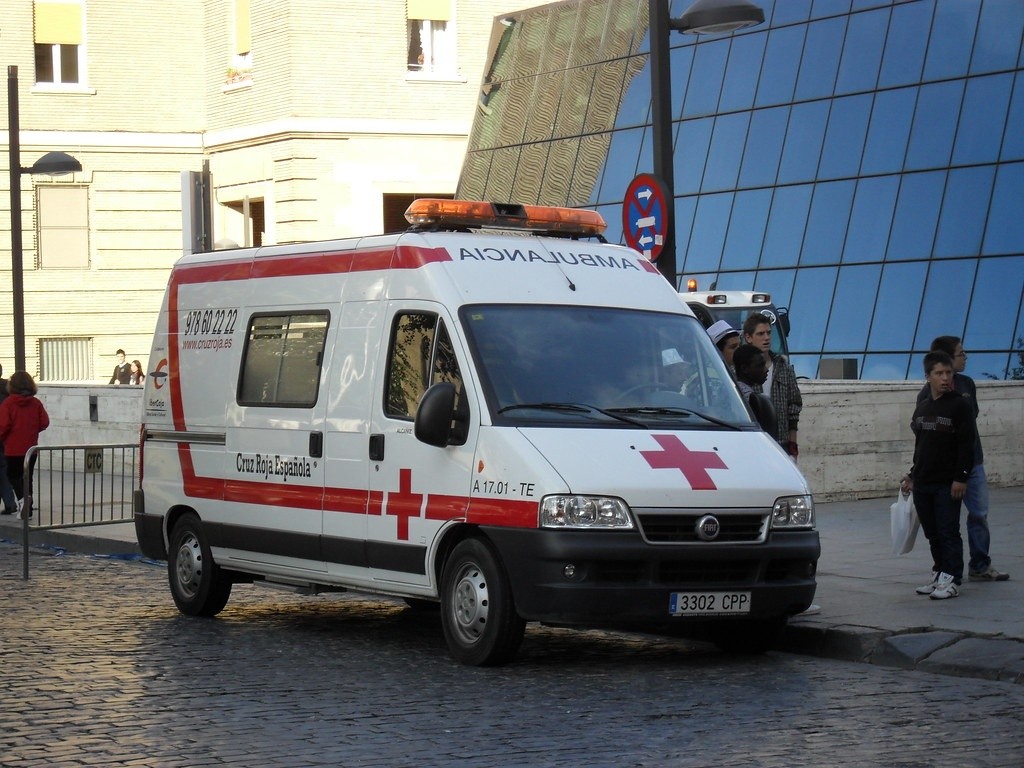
[16, 495, 32, 519]
[1, 507, 18, 515]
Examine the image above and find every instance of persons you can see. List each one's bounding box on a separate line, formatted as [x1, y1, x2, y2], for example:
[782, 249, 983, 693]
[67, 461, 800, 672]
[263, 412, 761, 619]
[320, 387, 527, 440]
[890, 336, 1010, 600]
[107, 349, 145, 386]
[660, 313, 802, 466]
[0, 364, 50, 520]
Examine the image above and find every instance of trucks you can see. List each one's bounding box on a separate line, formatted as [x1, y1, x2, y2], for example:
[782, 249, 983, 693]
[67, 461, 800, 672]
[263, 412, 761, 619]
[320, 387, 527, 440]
[662, 281, 794, 411]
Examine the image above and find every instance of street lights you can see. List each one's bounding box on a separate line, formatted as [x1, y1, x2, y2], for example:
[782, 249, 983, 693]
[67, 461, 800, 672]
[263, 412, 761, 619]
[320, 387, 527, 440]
[5, 64, 86, 378]
[643, 0, 768, 287]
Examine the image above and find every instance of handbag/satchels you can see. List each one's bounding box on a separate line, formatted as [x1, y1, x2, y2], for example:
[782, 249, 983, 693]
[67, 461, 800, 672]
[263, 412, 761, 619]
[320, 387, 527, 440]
[891, 485, 920, 557]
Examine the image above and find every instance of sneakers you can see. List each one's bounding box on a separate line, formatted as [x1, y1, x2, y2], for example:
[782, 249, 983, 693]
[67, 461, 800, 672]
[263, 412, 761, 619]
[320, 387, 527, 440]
[929, 571, 960, 599]
[798, 605, 821, 616]
[916, 569, 938, 594]
[969, 565, 1009, 583]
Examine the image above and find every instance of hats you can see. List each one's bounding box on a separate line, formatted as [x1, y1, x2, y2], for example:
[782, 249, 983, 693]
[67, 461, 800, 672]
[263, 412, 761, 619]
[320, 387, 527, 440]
[706, 320, 737, 345]
[660, 348, 692, 368]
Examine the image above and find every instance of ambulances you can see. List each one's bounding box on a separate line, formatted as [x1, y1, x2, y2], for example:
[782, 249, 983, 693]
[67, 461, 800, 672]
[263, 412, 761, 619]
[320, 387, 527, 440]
[130, 196, 828, 666]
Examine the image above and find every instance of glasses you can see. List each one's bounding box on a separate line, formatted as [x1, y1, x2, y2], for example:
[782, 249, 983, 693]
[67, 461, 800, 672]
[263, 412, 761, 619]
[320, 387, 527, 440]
[952, 351, 966, 359]
